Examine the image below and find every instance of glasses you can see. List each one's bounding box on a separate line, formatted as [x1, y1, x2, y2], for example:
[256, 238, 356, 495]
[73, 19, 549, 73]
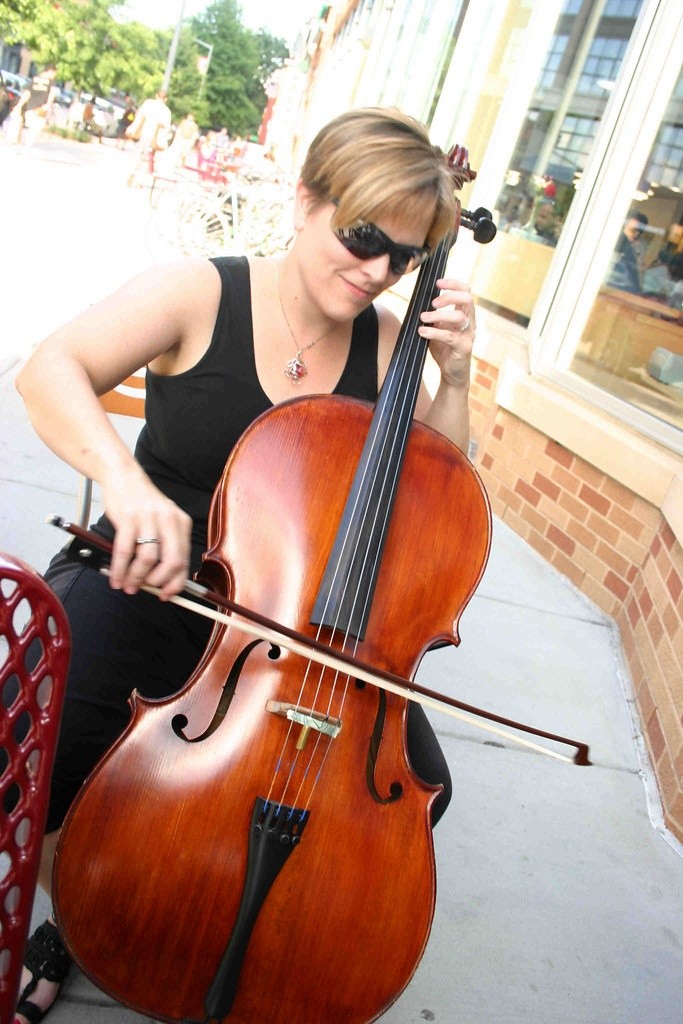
[312, 179, 430, 279]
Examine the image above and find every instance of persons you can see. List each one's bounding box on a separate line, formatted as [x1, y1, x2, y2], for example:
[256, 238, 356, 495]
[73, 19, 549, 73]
[607, 212, 683, 305]
[534, 172, 563, 247]
[0, 104, 471, 1024]
[0, 66, 251, 173]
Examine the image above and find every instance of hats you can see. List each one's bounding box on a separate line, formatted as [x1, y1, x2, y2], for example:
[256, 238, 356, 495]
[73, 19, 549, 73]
[89, 96, 97, 105]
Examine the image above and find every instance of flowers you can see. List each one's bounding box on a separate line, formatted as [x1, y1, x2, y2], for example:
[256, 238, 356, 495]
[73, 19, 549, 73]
[530, 176, 557, 205]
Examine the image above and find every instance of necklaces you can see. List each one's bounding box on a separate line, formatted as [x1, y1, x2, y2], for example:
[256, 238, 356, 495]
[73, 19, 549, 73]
[279, 291, 342, 380]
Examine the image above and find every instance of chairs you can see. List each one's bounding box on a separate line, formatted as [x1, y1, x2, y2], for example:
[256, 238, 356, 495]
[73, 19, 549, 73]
[0, 364, 148, 1024]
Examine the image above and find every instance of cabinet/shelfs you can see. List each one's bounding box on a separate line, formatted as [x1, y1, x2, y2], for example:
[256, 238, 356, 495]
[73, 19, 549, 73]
[475, 229, 683, 396]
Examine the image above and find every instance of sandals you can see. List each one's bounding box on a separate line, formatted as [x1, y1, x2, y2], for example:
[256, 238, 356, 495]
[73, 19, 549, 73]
[14, 911, 73, 1022]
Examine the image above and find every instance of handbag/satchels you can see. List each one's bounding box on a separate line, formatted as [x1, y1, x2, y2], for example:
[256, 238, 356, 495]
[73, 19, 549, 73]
[155, 126, 169, 151]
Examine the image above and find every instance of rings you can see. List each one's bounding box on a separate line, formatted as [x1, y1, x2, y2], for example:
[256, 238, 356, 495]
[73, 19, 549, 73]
[456, 318, 471, 332]
[138, 536, 160, 545]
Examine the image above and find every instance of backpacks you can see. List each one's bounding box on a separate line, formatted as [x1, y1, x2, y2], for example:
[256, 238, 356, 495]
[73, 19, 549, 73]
[123, 108, 134, 125]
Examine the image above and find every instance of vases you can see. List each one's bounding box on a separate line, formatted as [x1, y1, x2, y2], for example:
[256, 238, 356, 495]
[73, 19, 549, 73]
[523, 202, 542, 233]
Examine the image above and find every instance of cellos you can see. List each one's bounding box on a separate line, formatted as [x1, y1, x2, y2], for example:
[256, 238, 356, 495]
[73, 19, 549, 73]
[50, 138, 498, 1023]
